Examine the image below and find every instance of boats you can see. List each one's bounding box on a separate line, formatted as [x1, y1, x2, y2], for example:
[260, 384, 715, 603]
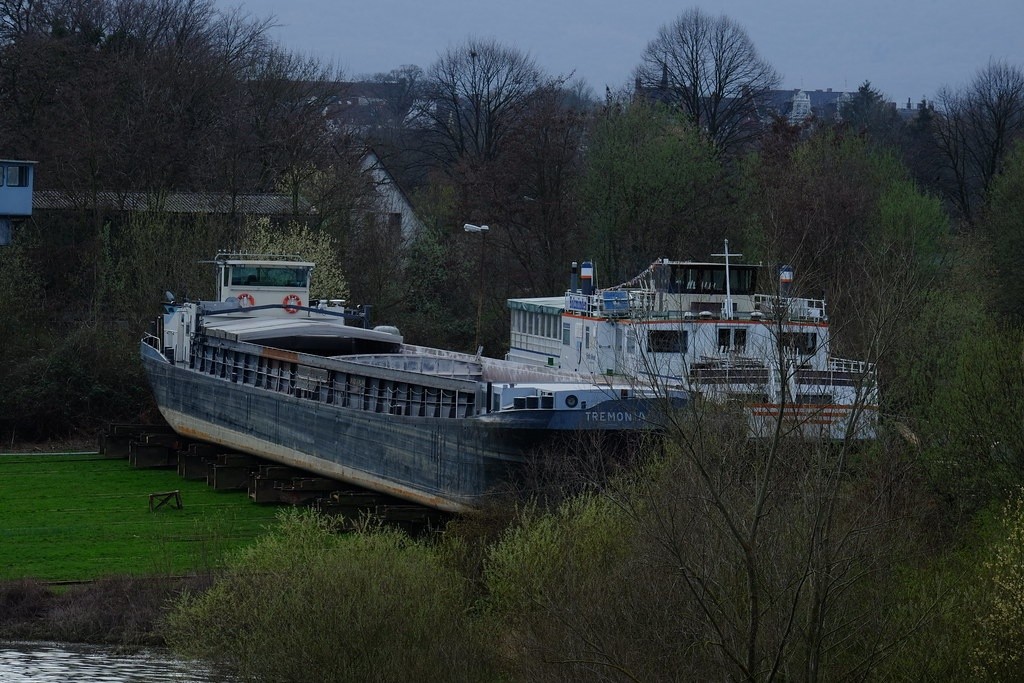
[502, 239, 878, 442]
[138, 252, 689, 522]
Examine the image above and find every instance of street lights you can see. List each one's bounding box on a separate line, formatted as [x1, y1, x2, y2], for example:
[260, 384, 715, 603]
[463, 224, 489, 356]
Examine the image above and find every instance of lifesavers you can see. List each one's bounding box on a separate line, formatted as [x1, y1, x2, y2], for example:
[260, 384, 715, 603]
[237, 293, 254, 313]
[283, 294, 301, 314]
